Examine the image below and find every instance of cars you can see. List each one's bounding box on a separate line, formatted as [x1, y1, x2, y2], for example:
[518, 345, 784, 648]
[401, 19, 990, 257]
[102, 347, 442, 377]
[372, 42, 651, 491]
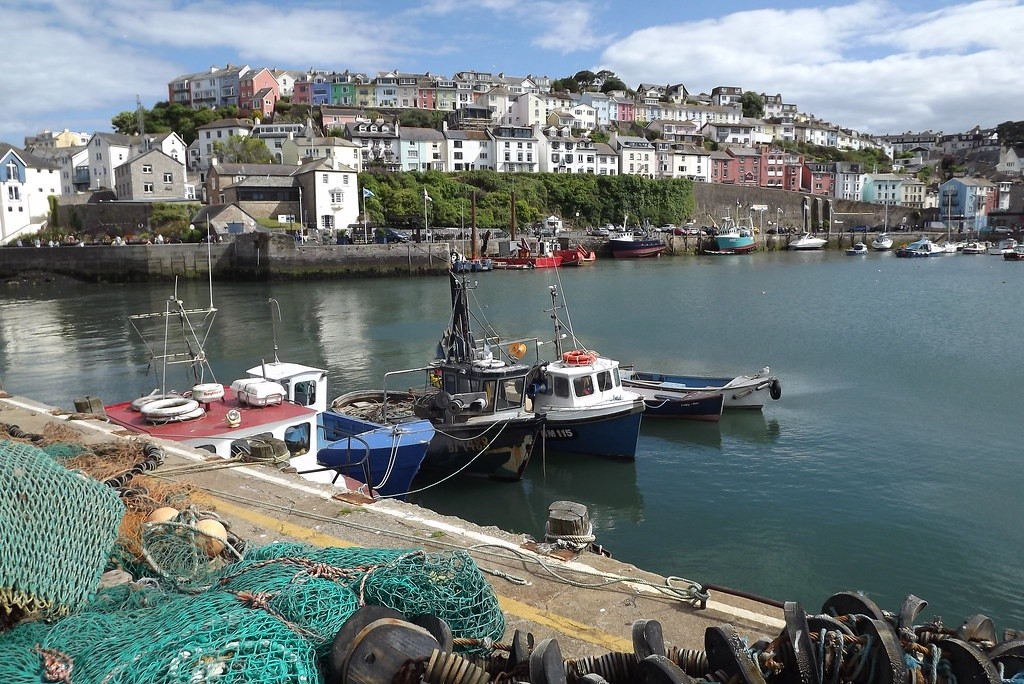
[602, 223, 615, 231]
[869, 224, 891, 233]
[651, 221, 715, 236]
[590, 227, 609, 237]
[375, 227, 413, 244]
[975, 225, 992, 235]
[993, 226, 1014, 234]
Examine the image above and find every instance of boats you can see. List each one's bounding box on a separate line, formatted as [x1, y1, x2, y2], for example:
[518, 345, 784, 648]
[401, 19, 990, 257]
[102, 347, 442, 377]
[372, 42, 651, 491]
[608, 214, 667, 260]
[632, 367, 785, 412]
[622, 385, 724, 423]
[705, 198, 758, 255]
[788, 230, 827, 250]
[895, 234, 946, 257]
[846, 241, 869, 255]
[98, 208, 644, 503]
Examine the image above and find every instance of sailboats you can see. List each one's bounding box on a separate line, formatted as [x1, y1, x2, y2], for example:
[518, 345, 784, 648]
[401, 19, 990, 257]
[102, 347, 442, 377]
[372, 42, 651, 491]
[941, 193, 1024, 261]
[869, 179, 899, 250]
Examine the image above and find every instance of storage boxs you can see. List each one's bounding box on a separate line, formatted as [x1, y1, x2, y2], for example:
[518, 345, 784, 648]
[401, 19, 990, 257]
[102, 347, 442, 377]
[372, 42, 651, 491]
[229, 377, 287, 405]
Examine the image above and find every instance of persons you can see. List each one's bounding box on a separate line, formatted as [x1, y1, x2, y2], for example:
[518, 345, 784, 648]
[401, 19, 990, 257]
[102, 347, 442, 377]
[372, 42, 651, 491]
[17, 232, 223, 247]
[344, 229, 350, 244]
[294, 227, 307, 242]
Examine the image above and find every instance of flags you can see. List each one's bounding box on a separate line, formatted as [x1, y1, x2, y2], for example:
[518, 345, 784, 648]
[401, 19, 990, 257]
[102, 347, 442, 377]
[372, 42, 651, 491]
[364, 189, 375, 197]
[425, 190, 433, 201]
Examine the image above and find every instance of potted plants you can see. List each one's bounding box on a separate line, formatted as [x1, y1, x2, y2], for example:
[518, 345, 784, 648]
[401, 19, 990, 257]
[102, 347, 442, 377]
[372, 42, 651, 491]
[337, 229, 348, 244]
[375, 228, 386, 244]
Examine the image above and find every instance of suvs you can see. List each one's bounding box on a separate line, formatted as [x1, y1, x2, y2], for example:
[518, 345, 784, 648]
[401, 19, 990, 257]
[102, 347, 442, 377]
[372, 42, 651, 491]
[848, 225, 869, 233]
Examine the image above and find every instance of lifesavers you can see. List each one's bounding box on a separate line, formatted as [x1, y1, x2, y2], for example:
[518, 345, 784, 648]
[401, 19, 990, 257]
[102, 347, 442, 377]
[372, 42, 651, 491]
[563, 351, 596, 365]
[131, 394, 202, 421]
